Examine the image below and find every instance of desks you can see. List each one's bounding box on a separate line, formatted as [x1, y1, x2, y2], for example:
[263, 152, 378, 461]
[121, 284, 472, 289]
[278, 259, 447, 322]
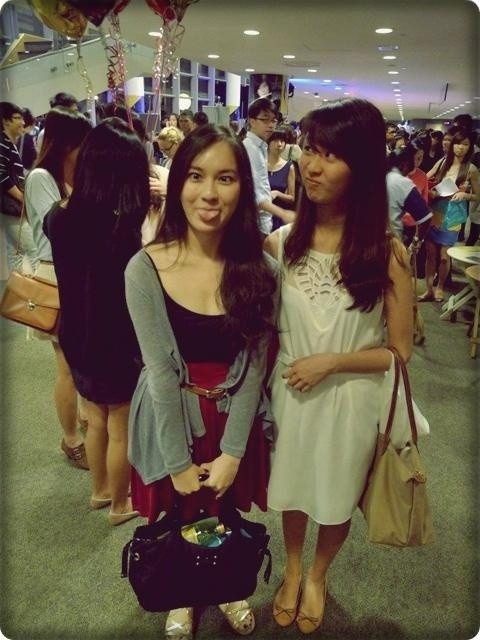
[440, 246, 479, 321]
[465, 265, 480, 360]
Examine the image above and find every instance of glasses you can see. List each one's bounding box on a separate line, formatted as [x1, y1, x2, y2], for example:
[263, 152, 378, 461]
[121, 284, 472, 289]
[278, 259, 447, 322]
[256, 118, 277, 124]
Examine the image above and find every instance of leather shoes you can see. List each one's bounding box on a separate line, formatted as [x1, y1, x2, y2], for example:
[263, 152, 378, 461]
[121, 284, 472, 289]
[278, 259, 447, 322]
[273, 570, 303, 627]
[90, 492, 140, 524]
[296, 573, 327, 635]
[61, 435, 90, 468]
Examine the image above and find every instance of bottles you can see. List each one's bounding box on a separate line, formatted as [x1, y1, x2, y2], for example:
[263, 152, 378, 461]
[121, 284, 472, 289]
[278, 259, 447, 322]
[177, 521, 226, 545]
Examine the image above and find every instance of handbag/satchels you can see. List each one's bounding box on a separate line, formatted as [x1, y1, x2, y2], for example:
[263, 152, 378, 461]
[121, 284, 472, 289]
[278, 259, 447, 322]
[121, 473, 271, 611]
[0, 272, 59, 333]
[358, 432, 436, 545]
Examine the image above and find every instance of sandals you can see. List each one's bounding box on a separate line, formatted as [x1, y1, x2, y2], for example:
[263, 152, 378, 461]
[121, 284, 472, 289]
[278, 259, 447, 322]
[165, 607, 194, 640]
[218, 600, 255, 634]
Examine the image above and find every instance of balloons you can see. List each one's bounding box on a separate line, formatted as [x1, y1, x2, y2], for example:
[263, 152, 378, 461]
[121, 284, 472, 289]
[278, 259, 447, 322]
[25, 0, 208, 145]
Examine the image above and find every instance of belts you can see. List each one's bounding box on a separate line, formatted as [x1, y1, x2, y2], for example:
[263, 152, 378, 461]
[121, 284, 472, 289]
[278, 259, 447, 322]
[182, 382, 228, 399]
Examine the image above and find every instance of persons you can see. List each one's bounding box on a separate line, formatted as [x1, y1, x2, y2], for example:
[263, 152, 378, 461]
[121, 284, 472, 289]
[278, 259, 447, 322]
[22, 104, 95, 473]
[1, 90, 478, 306]
[39, 113, 168, 529]
[259, 95, 433, 636]
[120, 120, 282, 639]
